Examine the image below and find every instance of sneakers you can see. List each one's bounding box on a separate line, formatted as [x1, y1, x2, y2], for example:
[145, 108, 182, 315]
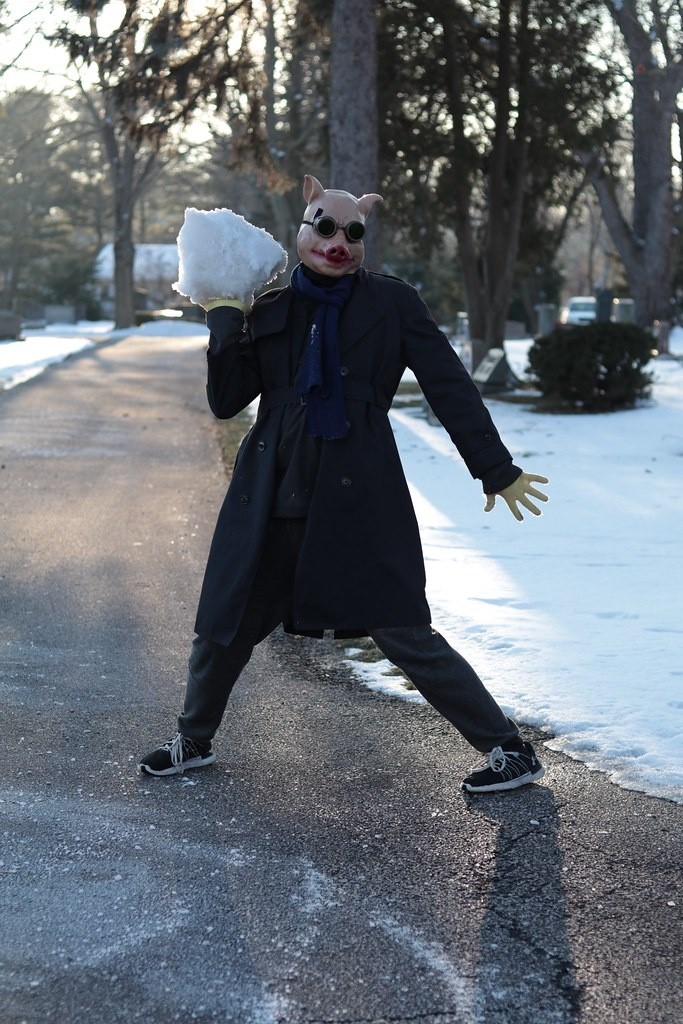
[138, 735, 218, 777]
[460, 741, 547, 794]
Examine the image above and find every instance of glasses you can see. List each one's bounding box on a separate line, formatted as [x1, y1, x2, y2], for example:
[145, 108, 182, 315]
[300, 213, 372, 243]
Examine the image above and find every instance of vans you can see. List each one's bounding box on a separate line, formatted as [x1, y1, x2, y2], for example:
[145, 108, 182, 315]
[561, 297, 634, 327]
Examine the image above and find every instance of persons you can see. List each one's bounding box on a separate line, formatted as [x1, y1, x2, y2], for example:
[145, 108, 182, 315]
[136, 173, 553, 793]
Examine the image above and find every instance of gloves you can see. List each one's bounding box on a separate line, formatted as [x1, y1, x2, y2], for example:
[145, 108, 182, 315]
[194, 294, 254, 314]
[481, 472, 552, 522]
